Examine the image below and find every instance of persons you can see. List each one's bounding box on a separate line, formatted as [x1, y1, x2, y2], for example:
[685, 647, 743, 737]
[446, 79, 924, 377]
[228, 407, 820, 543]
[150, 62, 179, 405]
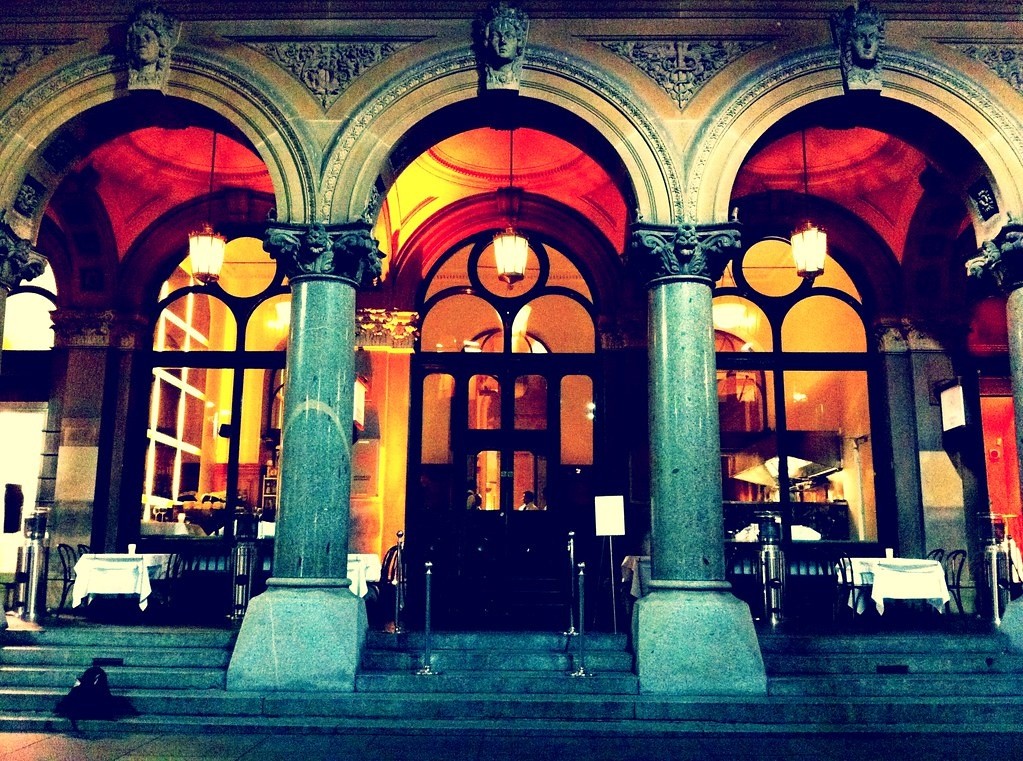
[484, 17, 524, 84]
[518, 491, 539, 510]
[841, 13, 885, 85]
[125, 19, 170, 85]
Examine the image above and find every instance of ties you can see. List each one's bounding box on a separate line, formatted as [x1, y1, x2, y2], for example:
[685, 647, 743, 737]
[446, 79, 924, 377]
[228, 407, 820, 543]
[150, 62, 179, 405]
[524, 506, 526, 510]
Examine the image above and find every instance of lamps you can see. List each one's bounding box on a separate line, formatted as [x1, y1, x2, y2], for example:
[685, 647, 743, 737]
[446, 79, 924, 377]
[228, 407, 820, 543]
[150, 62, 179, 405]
[491, 131, 531, 282]
[594, 496, 626, 635]
[188, 129, 224, 281]
[792, 129, 827, 277]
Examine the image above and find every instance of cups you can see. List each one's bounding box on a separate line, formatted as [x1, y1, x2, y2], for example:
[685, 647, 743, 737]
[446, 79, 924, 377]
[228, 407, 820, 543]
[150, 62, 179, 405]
[127, 544, 137, 553]
[885, 547, 894, 558]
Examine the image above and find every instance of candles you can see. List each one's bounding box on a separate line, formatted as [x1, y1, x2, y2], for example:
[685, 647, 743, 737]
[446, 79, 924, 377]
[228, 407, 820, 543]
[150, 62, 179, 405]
[885, 546, 893, 559]
[127, 543, 136, 554]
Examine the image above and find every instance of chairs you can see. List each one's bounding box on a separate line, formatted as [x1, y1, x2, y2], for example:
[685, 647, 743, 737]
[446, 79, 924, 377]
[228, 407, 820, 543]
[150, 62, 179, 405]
[56, 542, 77, 616]
[74, 545, 94, 607]
[148, 545, 194, 613]
[376, 546, 410, 630]
[921, 547, 967, 616]
[817, 547, 867, 621]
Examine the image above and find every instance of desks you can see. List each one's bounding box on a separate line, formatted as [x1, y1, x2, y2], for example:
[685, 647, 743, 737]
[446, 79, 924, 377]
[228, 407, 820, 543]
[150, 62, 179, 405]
[842, 556, 949, 628]
[347, 553, 379, 599]
[72, 552, 179, 614]
